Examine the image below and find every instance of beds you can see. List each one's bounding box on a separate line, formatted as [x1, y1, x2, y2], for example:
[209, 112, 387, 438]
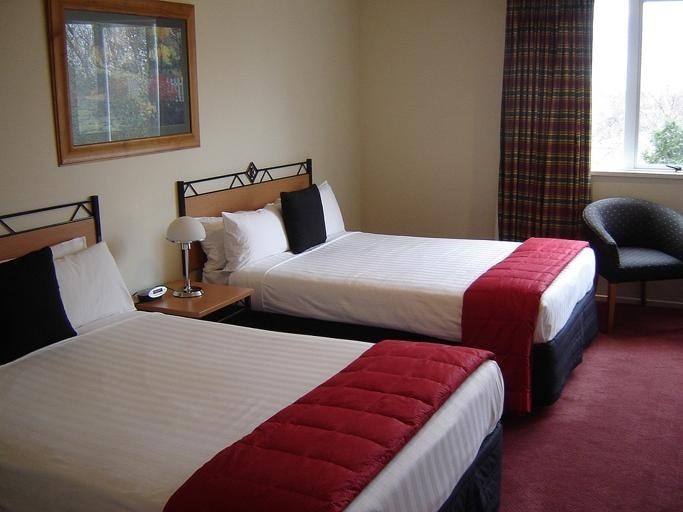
[176, 158, 597, 420]
[0, 196, 505, 512]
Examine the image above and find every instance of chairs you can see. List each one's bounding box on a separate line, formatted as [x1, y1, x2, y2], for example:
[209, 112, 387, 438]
[582, 196, 682, 336]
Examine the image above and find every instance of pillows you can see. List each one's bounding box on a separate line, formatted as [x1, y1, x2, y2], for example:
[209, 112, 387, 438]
[197, 181, 346, 273]
[0, 236, 137, 367]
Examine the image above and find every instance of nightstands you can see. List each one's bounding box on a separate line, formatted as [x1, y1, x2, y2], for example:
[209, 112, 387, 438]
[137, 279, 254, 324]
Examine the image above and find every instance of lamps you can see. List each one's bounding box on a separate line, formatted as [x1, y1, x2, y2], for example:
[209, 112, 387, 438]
[167, 216, 205, 297]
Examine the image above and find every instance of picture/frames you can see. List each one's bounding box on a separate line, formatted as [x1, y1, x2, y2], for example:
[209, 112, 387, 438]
[46, 0, 200, 166]
